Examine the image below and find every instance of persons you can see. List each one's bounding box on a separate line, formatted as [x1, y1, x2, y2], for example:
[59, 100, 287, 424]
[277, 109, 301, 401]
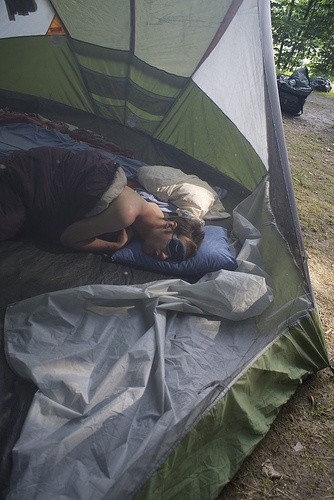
[0, 147, 206, 262]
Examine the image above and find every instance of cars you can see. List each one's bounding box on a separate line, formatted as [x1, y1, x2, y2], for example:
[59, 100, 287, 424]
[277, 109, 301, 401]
[311, 77, 332, 92]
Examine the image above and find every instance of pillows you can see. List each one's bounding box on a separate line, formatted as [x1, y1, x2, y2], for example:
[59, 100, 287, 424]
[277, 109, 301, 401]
[111, 225, 240, 276]
[136, 166, 232, 220]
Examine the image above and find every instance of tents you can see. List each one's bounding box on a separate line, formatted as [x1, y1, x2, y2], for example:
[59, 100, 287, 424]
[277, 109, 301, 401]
[0, 0, 334, 500]
